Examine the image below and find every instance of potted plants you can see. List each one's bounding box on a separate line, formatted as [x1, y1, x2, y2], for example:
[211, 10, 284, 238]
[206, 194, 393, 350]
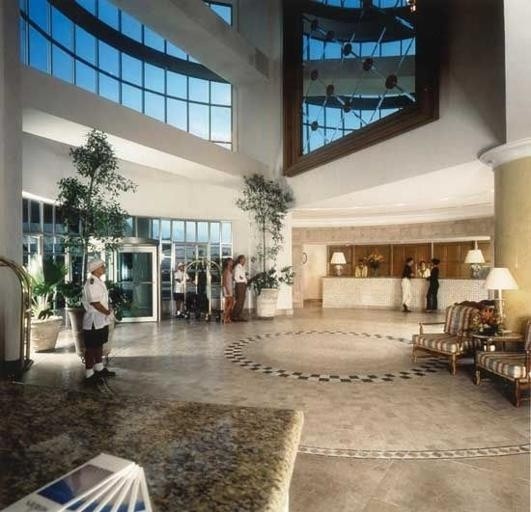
[21, 257, 65, 353]
[233, 170, 297, 320]
[55, 139, 138, 358]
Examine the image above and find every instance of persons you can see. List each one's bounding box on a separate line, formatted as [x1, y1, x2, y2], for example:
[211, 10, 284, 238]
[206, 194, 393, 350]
[354, 258, 368, 278]
[416, 261, 431, 278]
[173, 261, 191, 317]
[222, 258, 234, 323]
[81, 258, 116, 386]
[231, 254, 248, 321]
[400, 257, 414, 312]
[425, 257, 440, 313]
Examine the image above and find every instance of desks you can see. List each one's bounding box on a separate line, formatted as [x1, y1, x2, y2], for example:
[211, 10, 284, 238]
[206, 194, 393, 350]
[0, 378, 305, 512]
[473, 334, 524, 351]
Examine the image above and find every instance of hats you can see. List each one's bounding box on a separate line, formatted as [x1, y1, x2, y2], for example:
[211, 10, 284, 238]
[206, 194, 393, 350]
[88, 259, 104, 272]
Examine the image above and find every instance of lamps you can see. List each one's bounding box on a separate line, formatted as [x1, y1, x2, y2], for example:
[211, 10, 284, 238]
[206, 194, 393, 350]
[480, 267, 518, 316]
[464, 249, 485, 279]
[330, 251, 347, 276]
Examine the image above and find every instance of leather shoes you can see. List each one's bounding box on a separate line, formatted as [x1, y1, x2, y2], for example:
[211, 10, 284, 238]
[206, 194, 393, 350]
[95, 368, 115, 376]
[85, 373, 104, 384]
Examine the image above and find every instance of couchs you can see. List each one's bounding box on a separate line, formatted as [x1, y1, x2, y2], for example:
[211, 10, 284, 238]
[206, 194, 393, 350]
[471, 319, 531, 406]
[409, 301, 481, 374]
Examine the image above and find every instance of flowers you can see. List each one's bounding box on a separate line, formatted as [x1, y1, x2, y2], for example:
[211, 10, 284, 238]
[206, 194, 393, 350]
[363, 250, 384, 277]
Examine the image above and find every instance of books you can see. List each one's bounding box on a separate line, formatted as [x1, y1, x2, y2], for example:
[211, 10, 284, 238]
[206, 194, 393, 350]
[1, 450, 153, 511]
